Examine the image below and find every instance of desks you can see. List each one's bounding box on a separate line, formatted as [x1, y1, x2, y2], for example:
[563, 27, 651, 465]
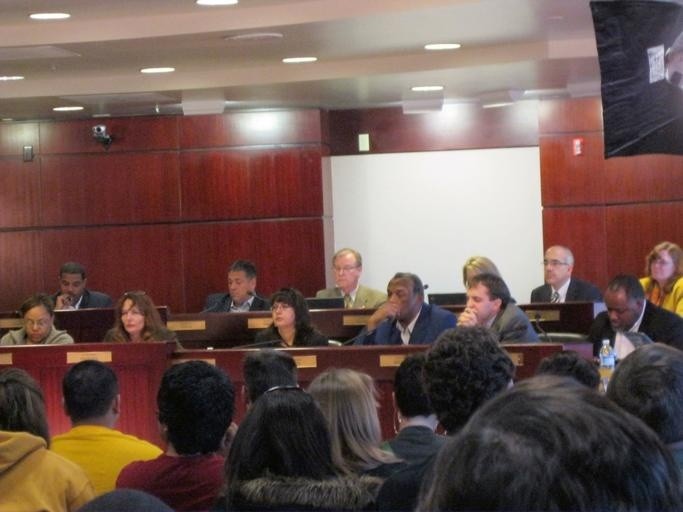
[0, 296, 602, 451]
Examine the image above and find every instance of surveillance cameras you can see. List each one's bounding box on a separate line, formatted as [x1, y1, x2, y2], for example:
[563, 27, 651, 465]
[92, 125, 106, 136]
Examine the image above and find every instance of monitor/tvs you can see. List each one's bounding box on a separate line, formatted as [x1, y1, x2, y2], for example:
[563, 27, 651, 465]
[304, 297, 344, 309]
[428, 293, 466, 304]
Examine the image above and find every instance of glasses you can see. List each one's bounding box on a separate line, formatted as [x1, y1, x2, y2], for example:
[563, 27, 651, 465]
[330, 263, 358, 272]
[270, 304, 292, 312]
[543, 260, 569, 267]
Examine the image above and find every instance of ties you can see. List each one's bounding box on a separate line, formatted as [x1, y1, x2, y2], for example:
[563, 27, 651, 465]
[343, 293, 351, 308]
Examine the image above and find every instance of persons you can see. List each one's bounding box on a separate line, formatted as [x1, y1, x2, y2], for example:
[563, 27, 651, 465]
[587, 274, 682, 354]
[0, 365, 92, 511]
[47, 360, 163, 492]
[43, 262, 111, 307]
[0, 292, 74, 345]
[316, 247, 388, 310]
[461, 256, 515, 307]
[253, 288, 328, 346]
[530, 246, 602, 302]
[636, 241, 683, 321]
[364, 272, 455, 344]
[455, 272, 540, 344]
[102, 290, 183, 350]
[205, 259, 269, 312]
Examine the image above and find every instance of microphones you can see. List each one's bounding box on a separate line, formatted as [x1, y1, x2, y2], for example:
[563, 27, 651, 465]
[198, 299, 222, 312]
[247, 290, 272, 306]
[341, 328, 377, 346]
[231, 338, 283, 348]
[535, 313, 549, 343]
[60, 295, 72, 309]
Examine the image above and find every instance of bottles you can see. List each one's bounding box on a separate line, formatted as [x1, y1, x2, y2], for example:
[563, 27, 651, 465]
[597, 338, 616, 391]
